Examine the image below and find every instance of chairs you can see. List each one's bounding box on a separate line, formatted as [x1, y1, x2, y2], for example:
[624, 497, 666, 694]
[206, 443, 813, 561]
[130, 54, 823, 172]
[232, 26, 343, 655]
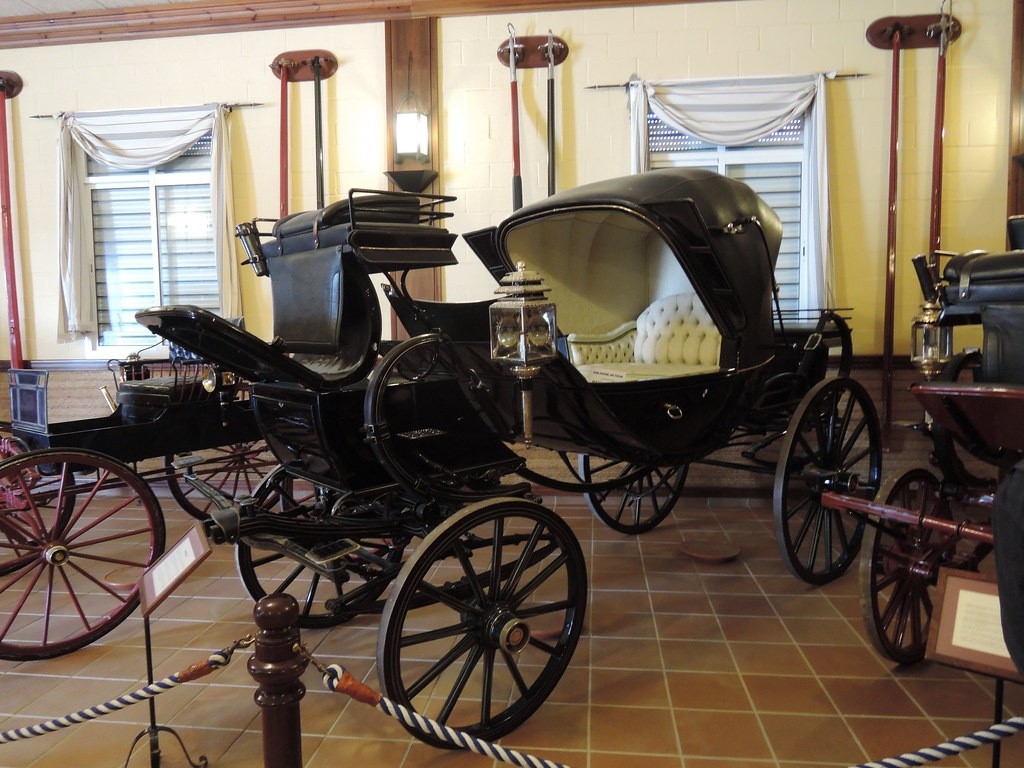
[912, 216, 1024, 387]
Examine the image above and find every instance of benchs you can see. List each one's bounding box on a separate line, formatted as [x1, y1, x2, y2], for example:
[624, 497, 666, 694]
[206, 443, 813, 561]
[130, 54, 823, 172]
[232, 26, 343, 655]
[565, 292, 737, 386]
[237, 187, 459, 354]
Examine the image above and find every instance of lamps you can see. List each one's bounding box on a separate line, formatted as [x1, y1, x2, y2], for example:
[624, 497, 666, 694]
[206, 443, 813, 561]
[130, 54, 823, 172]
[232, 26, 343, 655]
[392, 91, 433, 164]
